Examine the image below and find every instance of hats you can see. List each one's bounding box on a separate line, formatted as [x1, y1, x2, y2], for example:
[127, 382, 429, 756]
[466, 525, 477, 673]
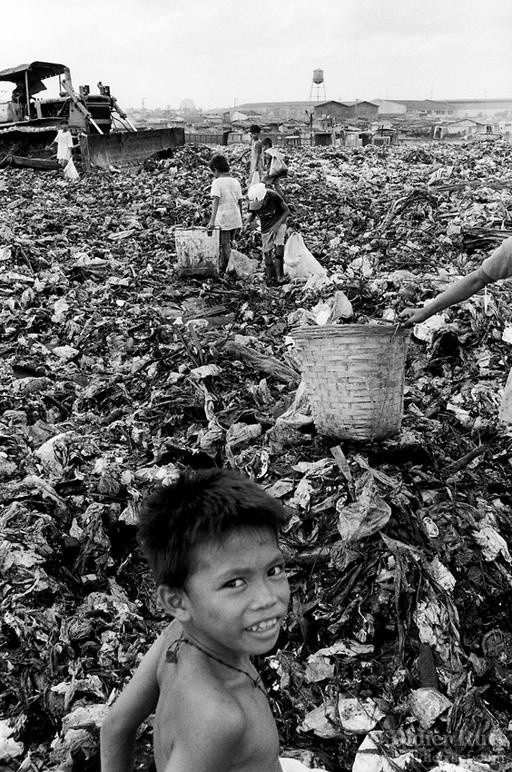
[246, 183, 266, 211]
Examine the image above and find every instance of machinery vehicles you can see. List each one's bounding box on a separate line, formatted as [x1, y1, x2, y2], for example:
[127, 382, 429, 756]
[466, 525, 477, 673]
[0, 61, 184, 174]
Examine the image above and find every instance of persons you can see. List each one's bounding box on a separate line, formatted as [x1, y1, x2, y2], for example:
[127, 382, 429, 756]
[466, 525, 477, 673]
[398, 235, 512, 425]
[207, 156, 243, 270]
[47, 120, 74, 168]
[239, 183, 290, 283]
[100, 470, 291, 772]
[249, 125, 288, 202]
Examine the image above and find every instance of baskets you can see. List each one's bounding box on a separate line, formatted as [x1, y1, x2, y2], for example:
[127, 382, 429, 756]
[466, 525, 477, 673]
[291, 323, 412, 441]
